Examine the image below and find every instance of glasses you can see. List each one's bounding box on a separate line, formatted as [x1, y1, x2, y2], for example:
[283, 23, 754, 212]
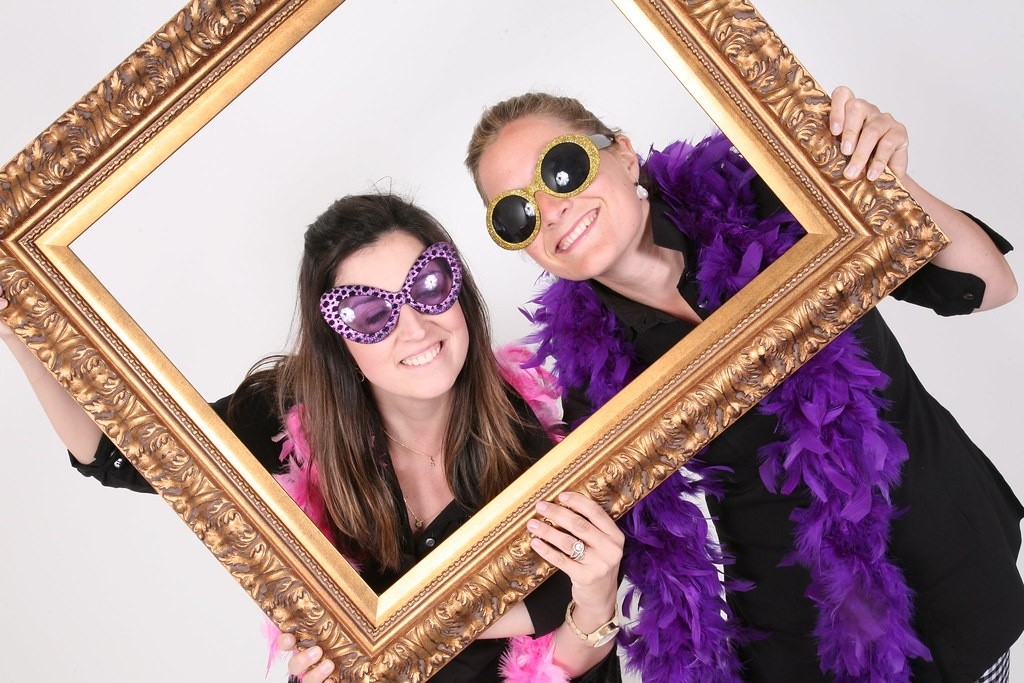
[486, 132, 616, 251]
[319, 241, 464, 344]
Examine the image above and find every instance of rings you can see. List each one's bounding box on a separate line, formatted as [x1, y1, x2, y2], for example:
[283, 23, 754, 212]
[570, 538, 590, 560]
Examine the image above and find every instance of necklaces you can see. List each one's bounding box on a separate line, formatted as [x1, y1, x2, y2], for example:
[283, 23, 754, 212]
[403, 495, 442, 529]
[373, 422, 440, 469]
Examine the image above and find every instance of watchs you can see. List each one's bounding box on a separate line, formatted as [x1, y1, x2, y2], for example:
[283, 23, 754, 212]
[565, 599, 620, 649]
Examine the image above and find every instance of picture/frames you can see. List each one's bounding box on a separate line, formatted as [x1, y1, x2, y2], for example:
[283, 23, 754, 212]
[0, 0, 951, 682]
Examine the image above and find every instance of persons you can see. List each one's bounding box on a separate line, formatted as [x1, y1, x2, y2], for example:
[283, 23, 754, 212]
[0, 197, 626, 683]
[462, 87, 1024, 683]
